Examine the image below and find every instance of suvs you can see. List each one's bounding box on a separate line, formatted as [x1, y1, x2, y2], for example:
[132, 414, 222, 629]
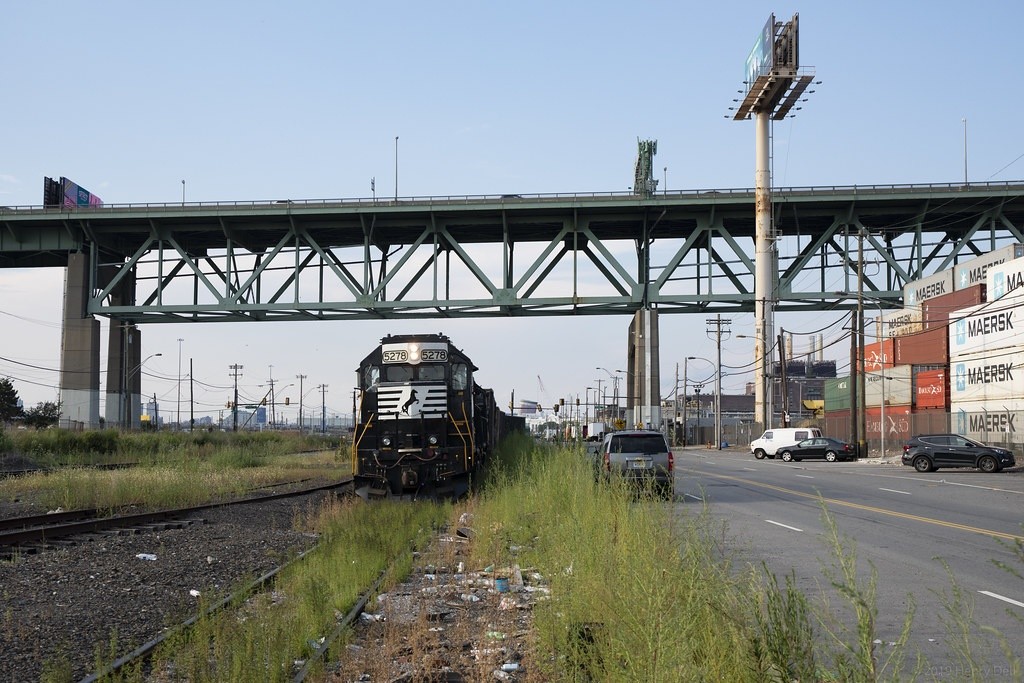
[901, 433, 1016, 473]
[587, 428, 674, 499]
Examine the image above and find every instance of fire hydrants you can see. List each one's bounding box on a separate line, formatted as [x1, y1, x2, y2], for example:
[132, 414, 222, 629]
[708, 441, 711, 449]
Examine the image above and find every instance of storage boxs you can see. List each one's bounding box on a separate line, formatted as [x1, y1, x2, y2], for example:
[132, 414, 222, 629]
[824, 243, 1024, 446]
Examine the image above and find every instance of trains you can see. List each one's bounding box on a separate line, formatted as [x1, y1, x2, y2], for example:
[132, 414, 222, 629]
[349, 333, 526, 501]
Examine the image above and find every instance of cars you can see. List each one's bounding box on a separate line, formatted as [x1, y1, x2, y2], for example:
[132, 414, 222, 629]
[777, 438, 856, 462]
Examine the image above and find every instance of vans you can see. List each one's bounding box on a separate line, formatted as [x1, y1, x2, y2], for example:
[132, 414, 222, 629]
[750, 427, 822, 459]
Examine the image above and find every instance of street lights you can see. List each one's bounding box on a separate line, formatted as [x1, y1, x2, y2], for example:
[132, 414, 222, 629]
[268, 365, 274, 425]
[688, 357, 718, 449]
[736, 335, 772, 430]
[273, 383, 294, 429]
[615, 370, 641, 423]
[122, 353, 163, 432]
[596, 367, 620, 420]
[300, 386, 321, 429]
[835, 291, 886, 459]
[177, 338, 184, 429]
